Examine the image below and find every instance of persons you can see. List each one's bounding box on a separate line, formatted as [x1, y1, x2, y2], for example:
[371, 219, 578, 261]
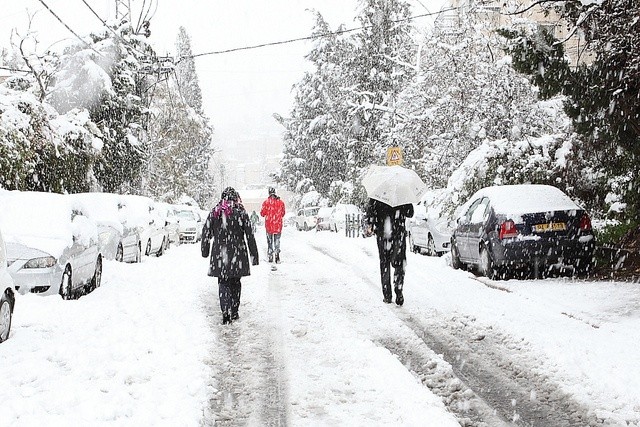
[200, 186, 259, 326]
[360, 198, 414, 307]
[261, 189, 286, 265]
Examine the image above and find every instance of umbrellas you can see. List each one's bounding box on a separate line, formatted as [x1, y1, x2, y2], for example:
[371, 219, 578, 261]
[361, 166, 429, 206]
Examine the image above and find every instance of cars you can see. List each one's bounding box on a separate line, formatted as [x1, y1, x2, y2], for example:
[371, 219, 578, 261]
[316, 207, 333, 231]
[405, 188, 452, 257]
[1, 192, 102, 299]
[0, 231, 16, 343]
[329, 204, 363, 233]
[120, 191, 204, 257]
[69, 192, 141, 263]
[451, 184, 595, 280]
[296, 207, 321, 232]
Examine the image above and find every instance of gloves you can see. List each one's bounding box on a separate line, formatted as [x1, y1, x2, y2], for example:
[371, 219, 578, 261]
[202, 251, 208, 257]
[251, 257, 259, 265]
